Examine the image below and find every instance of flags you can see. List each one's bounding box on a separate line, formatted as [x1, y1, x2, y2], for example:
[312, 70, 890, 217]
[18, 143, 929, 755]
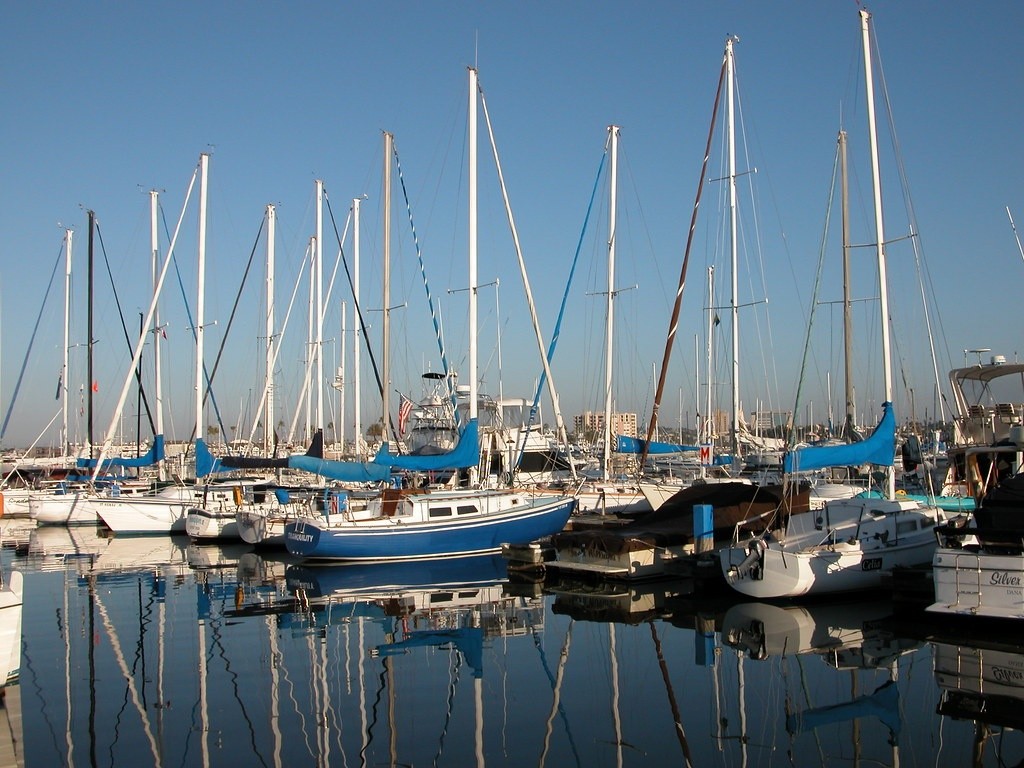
[162, 329, 167, 340]
[55, 376, 98, 415]
[397, 394, 414, 438]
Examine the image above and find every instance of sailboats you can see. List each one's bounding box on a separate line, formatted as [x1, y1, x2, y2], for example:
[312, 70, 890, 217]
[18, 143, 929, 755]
[1, 521, 1024, 768]
[2, 7, 1024, 604]
